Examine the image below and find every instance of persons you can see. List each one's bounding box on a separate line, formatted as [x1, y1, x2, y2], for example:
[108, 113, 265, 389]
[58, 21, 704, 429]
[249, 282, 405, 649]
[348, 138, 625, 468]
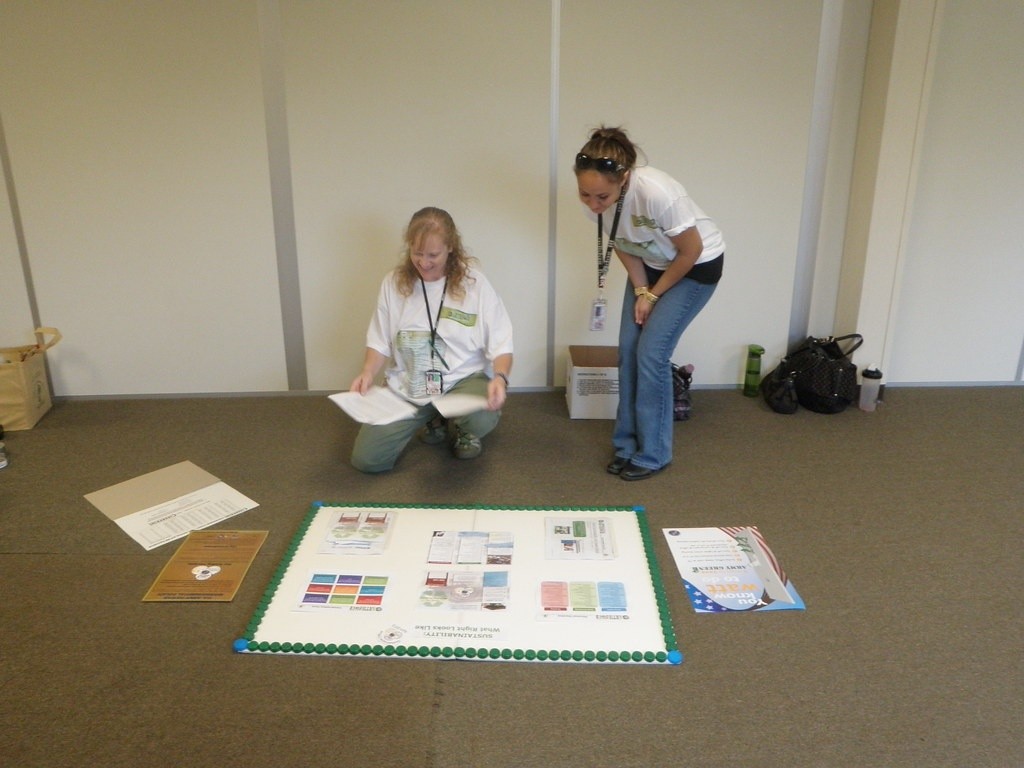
[428, 375, 434, 383]
[350, 206, 513, 472]
[574, 126, 727, 480]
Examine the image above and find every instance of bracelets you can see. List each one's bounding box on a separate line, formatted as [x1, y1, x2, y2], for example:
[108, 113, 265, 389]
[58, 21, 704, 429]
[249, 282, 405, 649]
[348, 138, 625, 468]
[495, 372, 509, 389]
[635, 287, 648, 296]
[644, 290, 658, 305]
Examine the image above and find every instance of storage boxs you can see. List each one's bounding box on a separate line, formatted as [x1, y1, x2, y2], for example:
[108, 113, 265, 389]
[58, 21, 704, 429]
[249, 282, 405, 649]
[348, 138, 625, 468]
[0, 346, 53, 432]
[566, 345, 620, 420]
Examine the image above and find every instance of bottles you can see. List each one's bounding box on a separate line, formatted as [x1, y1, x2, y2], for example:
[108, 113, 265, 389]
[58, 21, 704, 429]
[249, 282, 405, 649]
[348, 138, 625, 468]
[743, 344, 765, 398]
[859, 363, 882, 411]
[678, 364, 694, 380]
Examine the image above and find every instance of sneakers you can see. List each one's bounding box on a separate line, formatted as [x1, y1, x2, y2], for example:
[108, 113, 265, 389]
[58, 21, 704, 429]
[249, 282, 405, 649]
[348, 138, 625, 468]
[420, 412, 448, 444]
[454, 425, 482, 460]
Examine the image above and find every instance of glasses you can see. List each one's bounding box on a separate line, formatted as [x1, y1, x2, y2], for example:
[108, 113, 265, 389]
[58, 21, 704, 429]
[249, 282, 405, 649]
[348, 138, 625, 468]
[575, 154, 629, 173]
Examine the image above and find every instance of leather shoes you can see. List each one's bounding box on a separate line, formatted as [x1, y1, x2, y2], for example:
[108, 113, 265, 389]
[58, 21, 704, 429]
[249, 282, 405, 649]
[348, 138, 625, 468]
[607, 456, 669, 480]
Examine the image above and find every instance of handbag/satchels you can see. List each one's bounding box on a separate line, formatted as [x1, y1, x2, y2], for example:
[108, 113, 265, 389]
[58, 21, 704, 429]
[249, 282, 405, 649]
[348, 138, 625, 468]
[0, 328, 61, 432]
[760, 334, 863, 415]
[672, 362, 694, 422]
